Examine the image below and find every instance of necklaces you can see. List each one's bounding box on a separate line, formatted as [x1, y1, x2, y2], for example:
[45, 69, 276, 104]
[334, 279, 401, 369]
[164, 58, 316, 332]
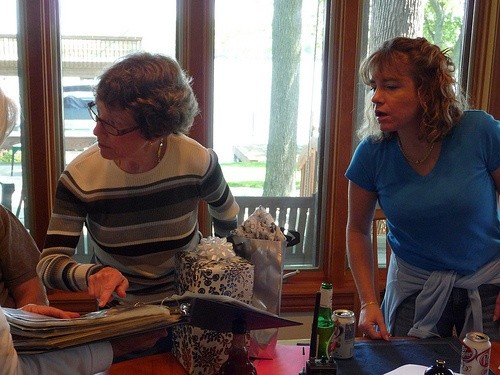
[118, 137, 164, 168]
[397, 135, 437, 165]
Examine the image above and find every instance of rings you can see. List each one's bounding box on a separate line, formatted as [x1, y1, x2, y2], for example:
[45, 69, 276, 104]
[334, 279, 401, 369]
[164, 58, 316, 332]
[363, 332, 369, 336]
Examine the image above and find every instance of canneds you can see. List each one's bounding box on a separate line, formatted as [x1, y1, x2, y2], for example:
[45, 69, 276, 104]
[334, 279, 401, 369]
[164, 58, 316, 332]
[331, 309, 355, 359]
[460, 331, 491, 375]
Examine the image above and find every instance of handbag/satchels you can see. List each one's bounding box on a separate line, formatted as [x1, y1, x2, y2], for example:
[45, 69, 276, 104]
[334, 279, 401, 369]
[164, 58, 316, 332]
[230, 228, 301, 360]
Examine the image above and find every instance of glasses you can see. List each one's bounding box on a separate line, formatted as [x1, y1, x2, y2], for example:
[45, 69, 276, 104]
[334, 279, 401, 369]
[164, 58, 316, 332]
[87, 100, 140, 136]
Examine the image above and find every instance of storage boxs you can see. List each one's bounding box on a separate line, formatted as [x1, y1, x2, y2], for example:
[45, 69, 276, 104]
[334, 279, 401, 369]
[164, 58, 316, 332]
[173, 261, 255, 375]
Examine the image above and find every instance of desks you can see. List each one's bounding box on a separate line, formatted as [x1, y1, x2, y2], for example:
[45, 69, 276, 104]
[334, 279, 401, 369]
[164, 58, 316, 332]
[87, 334, 500, 375]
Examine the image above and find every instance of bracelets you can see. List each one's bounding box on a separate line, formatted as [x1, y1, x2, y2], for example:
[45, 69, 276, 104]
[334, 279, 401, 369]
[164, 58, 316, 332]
[360, 301, 379, 309]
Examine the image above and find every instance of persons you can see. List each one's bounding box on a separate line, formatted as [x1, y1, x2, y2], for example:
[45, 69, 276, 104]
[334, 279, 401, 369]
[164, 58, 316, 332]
[344, 37, 500, 341]
[0, 89, 169, 375]
[36, 52, 239, 362]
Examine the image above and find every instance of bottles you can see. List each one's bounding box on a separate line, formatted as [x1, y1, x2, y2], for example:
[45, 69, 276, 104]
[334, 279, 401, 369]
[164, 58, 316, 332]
[423, 360, 455, 375]
[310, 282, 335, 360]
